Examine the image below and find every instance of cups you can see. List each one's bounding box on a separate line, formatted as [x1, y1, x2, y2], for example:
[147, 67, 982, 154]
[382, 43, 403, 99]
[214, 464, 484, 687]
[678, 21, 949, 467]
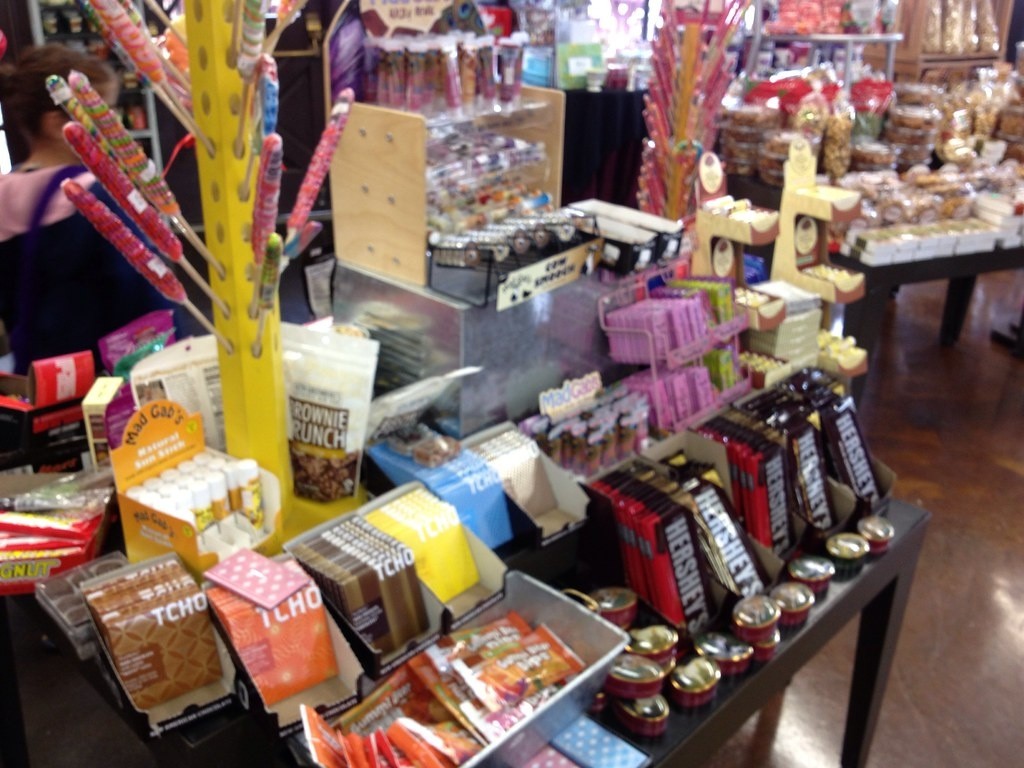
[584, 66, 607, 92]
[606, 64, 630, 90]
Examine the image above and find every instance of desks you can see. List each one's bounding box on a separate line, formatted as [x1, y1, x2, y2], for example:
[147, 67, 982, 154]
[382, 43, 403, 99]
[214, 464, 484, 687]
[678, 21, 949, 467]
[4, 499, 933, 768]
[563, 70, 648, 208]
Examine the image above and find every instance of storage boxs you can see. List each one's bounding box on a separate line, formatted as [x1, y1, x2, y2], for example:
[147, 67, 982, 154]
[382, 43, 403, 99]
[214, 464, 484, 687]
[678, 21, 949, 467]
[0, 350, 94, 474]
[327, 260, 609, 438]
[328, 81, 566, 288]
[286, 570, 631, 768]
[860, 0, 1013, 85]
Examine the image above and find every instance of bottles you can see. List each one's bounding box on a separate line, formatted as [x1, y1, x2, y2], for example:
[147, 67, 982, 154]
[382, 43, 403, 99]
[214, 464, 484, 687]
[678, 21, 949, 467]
[724, 21, 746, 76]
[756, 30, 775, 78]
[852, 42, 864, 69]
[833, 45, 845, 76]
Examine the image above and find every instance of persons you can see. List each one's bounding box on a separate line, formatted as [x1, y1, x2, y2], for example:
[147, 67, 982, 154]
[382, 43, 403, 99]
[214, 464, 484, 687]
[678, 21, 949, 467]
[0, 43, 186, 378]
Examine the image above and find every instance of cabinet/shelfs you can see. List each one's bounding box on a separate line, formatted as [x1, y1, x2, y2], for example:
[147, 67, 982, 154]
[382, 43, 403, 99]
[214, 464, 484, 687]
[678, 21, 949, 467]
[719, 33, 1024, 408]
